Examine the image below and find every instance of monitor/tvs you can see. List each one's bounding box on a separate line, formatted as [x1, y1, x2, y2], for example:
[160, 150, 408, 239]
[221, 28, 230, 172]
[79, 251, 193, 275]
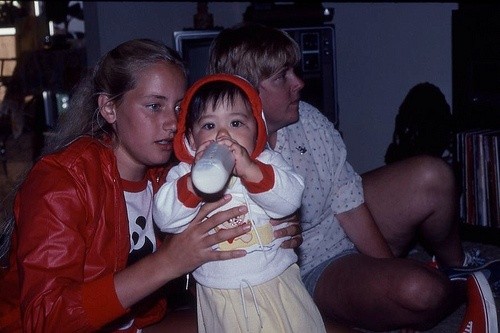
[174, 25, 339, 127]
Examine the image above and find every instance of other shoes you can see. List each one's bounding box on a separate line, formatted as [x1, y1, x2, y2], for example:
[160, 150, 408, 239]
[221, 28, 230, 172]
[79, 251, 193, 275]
[457, 270, 499, 333]
[432, 248, 499, 297]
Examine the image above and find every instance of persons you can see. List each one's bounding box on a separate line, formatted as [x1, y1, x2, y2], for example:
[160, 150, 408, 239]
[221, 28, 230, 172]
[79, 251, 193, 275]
[153, 74, 326, 332]
[0, 39, 500, 333]
[207, 24, 500, 327]
[0, 0, 87, 133]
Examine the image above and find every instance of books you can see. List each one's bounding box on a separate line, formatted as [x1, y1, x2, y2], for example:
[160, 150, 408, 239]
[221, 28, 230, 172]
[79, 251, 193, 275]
[457, 130, 500, 229]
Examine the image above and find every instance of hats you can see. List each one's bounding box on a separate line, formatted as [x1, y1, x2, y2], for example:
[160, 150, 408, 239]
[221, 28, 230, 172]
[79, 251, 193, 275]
[173, 73, 267, 164]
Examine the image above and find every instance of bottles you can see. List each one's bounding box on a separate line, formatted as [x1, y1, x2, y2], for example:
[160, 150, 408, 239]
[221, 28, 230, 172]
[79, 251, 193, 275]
[191, 141, 235, 195]
